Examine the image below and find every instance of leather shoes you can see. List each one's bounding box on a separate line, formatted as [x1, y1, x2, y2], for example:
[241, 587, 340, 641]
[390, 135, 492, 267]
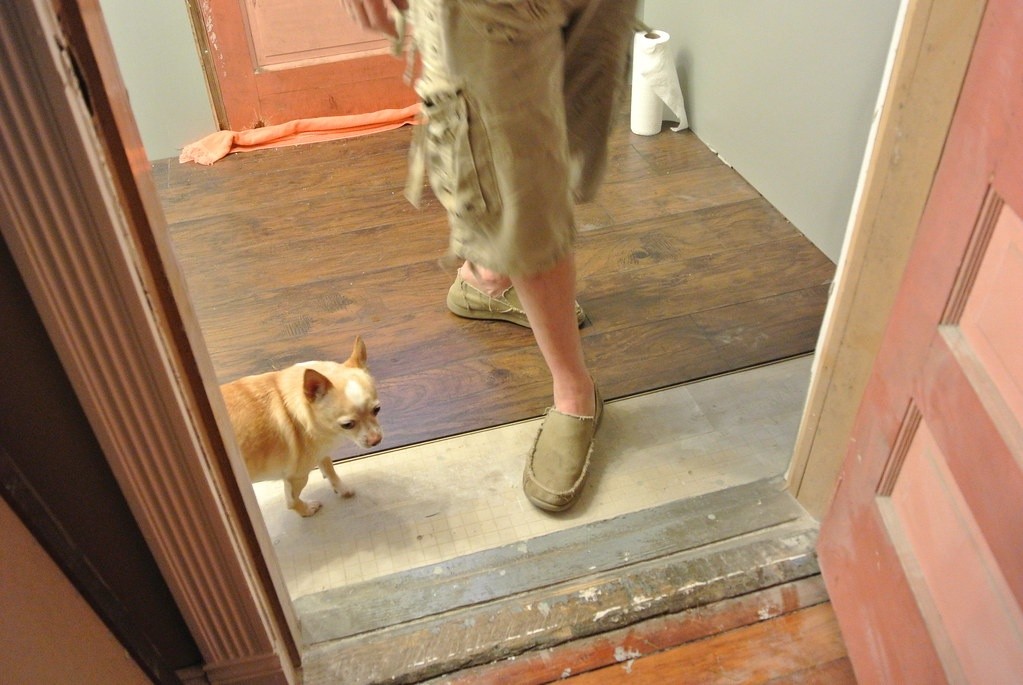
[448, 276, 584, 331]
[526, 387, 610, 512]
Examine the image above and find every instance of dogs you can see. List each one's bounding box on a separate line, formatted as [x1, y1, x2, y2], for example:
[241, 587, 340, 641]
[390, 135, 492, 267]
[219, 334, 382, 518]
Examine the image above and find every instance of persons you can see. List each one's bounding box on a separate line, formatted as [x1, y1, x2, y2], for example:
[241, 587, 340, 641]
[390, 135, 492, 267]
[338, 1, 653, 512]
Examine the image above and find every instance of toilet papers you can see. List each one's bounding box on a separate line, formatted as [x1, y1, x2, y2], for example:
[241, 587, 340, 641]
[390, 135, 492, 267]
[629, 29, 690, 137]
